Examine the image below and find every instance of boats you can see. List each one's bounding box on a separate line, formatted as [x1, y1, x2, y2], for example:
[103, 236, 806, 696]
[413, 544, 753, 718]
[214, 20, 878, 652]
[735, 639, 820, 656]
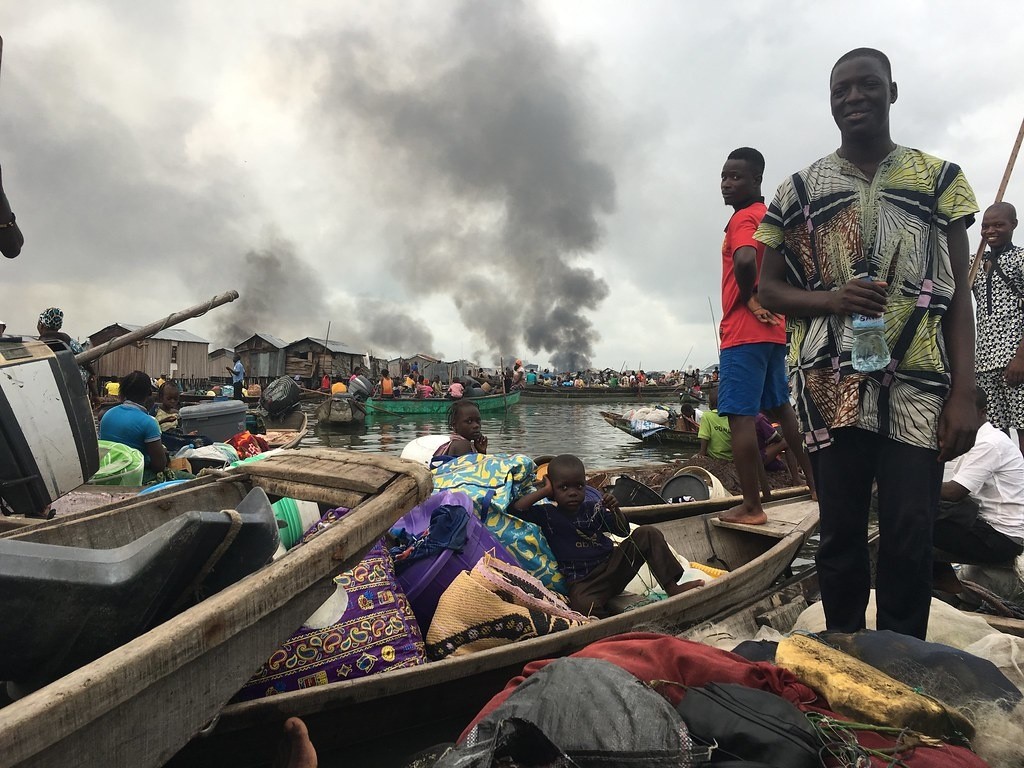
[472, 377, 711, 402]
[0, 396, 1024, 768]
[365, 390, 522, 416]
[314, 392, 366, 424]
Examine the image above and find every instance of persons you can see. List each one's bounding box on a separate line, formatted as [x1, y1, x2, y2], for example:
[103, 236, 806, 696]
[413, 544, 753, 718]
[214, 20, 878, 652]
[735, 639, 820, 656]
[149, 381, 184, 434]
[445, 400, 488, 458]
[505, 360, 719, 391]
[380, 369, 474, 399]
[226, 354, 245, 399]
[697, 389, 733, 462]
[99, 370, 167, 472]
[505, 455, 705, 620]
[755, 413, 805, 486]
[719, 148, 817, 525]
[156, 373, 167, 387]
[968, 202, 1024, 455]
[478, 368, 486, 378]
[104, 376, 120, 396]
[682, 404, 703, 423]
[37, 308, 101, 410]
[412, 361, 420, 376]
[932, 385, 1024, 593]
[349, 367, 364, 383]
[331, 377, 346, 395]
[750, 48, 979, 642]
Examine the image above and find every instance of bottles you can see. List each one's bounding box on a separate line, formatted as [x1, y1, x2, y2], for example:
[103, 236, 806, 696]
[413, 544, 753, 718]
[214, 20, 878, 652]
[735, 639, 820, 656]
[851, 277, 891, 371]
[173, 444, 194, 460]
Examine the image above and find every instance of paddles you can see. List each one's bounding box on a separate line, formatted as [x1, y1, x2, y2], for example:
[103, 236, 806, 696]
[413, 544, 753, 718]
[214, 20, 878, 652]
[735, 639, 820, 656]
[500, 356, 508, 410]
[226, 367, 246, 398]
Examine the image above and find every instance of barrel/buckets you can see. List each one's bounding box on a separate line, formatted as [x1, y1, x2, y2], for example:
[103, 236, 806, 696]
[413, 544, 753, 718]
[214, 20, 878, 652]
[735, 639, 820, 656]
[271, 495, 321, 550]
[674, 466, 733, 499]
[88, 438, 146, 487]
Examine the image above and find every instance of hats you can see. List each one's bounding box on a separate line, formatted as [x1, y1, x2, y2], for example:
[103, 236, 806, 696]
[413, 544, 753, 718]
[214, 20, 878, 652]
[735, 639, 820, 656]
[516, 360, 522, 365]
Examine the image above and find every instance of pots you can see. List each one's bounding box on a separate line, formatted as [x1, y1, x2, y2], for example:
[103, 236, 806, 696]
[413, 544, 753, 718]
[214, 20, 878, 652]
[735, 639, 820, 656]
[613, 474, 668, 505]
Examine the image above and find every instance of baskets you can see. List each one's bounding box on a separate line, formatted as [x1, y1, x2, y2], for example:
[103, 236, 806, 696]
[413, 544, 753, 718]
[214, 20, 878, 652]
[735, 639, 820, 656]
[586, 474, 611, 492]
